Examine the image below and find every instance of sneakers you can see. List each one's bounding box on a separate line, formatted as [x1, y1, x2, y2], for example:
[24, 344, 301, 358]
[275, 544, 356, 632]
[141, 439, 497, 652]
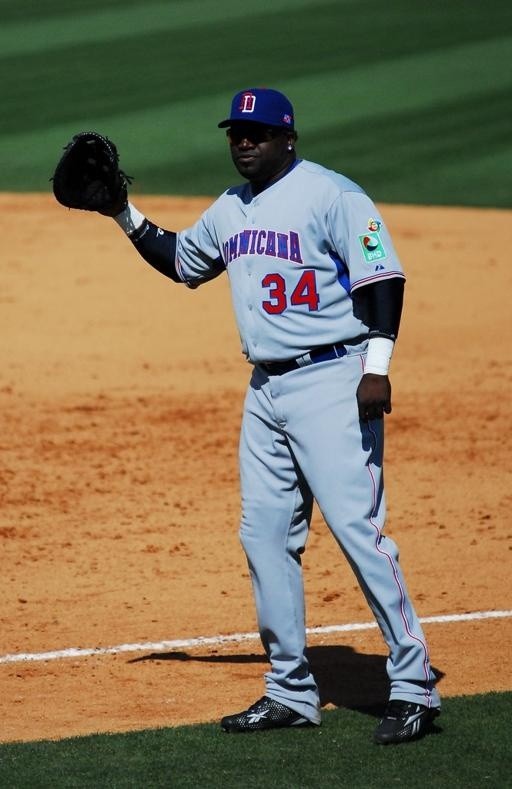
[372, 696, 441, 745]
[220, 693, 323, 732]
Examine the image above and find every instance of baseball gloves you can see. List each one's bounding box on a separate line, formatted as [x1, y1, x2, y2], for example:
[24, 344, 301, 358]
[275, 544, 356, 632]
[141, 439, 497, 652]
[49, 132, 133, 216]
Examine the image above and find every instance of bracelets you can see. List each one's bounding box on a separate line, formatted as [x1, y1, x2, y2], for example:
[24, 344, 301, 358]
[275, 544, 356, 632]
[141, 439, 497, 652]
[112, 201, 146, 238]
[364, 337, 394, 377]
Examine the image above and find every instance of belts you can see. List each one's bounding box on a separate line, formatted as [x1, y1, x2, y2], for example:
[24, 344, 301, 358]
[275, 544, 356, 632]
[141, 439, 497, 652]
[258, 342, 348, 376]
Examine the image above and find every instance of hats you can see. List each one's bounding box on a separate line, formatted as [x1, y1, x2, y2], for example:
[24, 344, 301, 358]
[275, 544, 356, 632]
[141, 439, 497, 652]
[218, 87, 295, 132]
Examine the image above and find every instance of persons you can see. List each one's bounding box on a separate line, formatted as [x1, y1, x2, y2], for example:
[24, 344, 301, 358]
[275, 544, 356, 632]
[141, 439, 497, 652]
[54, 89, 445, 744]
